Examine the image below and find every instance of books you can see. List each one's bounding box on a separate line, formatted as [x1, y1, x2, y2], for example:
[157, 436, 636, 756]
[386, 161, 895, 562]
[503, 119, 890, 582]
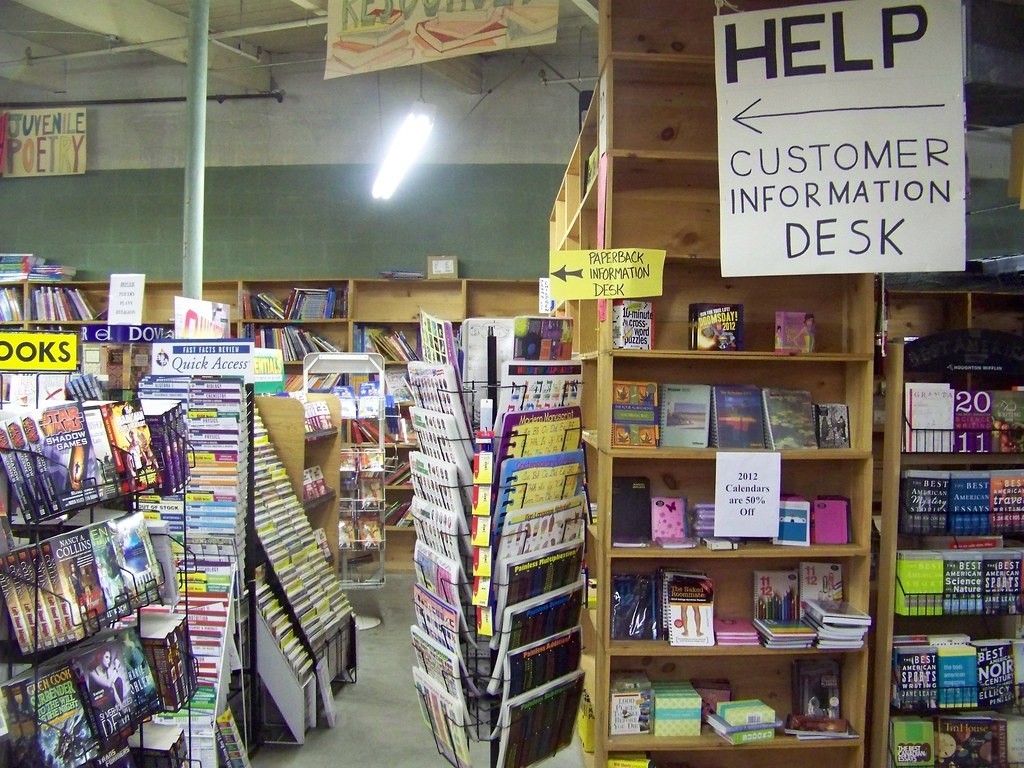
[762, 390, 822, 451]
[711, 385, 767, 449]
[612, 381, 659, 446]
[885, 381, 1024, 768]
[811, 402, 854, 449]
[606, 497, 871, 768]
[0, 251, 596, 768]
[661, 383, 711, 449]
[689, 303, 744, 352]
[612, 475, 653, 549]
[776, 310, 815, 354]
[614, 298, 653, 350]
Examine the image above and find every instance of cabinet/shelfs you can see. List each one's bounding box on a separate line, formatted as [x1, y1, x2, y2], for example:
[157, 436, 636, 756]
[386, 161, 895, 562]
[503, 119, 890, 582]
[0, 49, 1024, 768]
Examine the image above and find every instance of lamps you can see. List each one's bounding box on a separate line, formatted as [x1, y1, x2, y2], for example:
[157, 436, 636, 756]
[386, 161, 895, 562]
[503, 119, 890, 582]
[370, 64, 437, 200]
